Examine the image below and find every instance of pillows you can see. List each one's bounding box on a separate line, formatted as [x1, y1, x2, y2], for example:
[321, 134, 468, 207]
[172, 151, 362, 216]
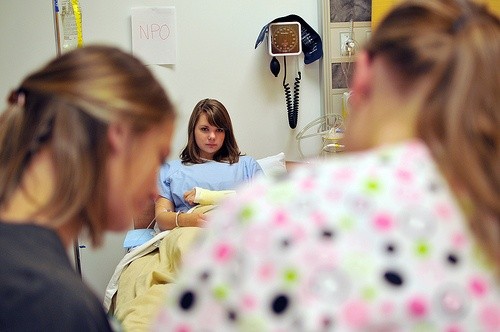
[255, 152, 287, 179]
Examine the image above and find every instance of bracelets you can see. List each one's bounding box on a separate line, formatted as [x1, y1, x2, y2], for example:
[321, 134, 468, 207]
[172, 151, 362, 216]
[176, 211, 180, 228]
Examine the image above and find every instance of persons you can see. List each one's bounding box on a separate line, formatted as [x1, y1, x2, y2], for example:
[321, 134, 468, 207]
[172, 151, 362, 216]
[152, 99, 265, 280]
[0, 45, 175, 332]
[158, 0, 500, 329]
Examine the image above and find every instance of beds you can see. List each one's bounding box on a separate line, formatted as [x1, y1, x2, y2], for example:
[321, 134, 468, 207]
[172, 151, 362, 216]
[103, 229, 221, 332]
